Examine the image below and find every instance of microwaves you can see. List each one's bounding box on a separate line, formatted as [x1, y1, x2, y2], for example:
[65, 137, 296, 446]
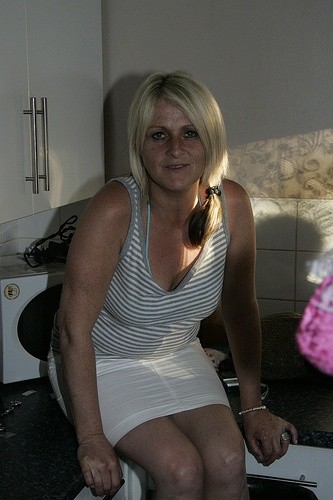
[0, 265, 66, 384]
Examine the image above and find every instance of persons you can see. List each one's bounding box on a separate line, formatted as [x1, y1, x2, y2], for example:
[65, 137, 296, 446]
[47, 69, 299, 500]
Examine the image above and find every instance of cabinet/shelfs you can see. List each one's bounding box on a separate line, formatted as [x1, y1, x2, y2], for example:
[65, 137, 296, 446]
[0, 1, 105, 225]
[101, 0, 332, 179]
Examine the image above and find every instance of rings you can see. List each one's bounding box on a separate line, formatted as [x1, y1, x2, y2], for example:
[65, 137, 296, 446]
[281, 431, 292, 442]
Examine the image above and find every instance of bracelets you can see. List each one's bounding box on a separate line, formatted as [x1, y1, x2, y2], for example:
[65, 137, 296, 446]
[238, 405, 267, 418]
